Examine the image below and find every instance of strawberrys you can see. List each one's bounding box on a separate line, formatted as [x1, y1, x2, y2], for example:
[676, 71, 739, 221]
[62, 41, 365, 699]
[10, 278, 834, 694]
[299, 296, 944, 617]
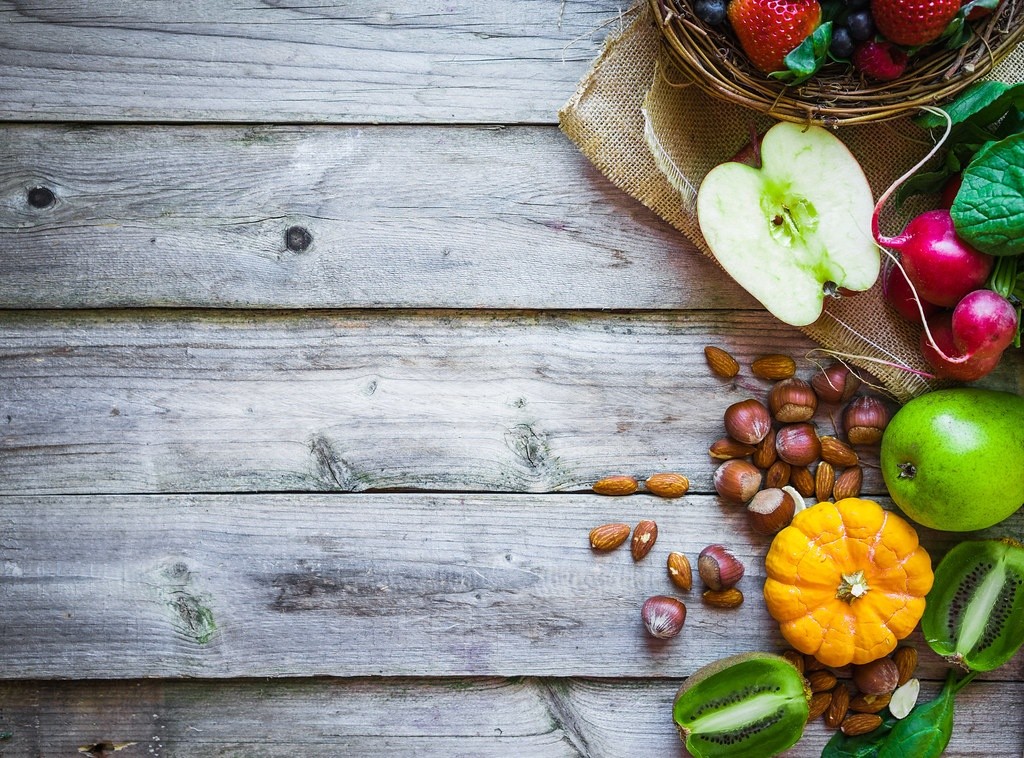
[726, 1, 961, 77]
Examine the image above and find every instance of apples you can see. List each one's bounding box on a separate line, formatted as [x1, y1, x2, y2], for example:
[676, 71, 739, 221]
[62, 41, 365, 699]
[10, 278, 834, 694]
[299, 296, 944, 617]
[695, 121, 882, 327]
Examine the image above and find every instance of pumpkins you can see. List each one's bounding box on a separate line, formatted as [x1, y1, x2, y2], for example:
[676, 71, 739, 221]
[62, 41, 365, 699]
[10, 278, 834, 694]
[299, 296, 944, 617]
[762, 499, 930, 667]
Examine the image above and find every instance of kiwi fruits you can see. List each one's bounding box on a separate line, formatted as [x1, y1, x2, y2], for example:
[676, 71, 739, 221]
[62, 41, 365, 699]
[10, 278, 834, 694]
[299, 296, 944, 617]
[921, 539, 1024, 672]
[672, 651, 812, 758]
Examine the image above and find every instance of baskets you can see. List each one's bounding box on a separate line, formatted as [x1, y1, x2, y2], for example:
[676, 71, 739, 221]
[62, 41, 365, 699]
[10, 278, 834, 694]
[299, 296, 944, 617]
[651, 1, 1024, 129]
